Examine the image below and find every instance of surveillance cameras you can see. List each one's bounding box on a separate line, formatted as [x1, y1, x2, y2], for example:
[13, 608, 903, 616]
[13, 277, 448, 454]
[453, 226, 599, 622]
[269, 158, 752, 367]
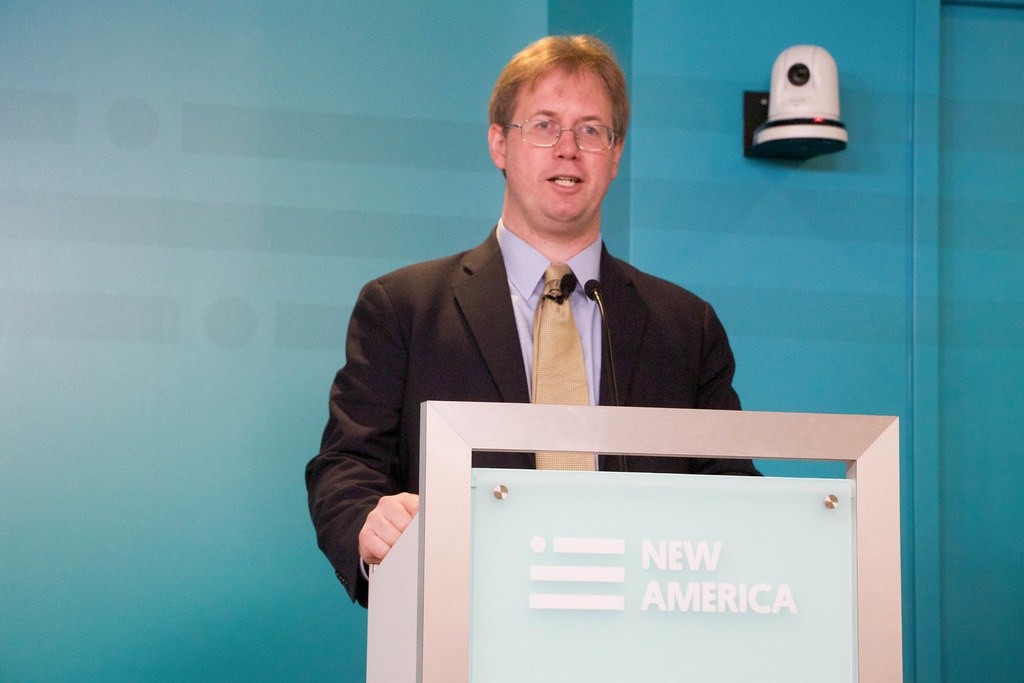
[766, 44, 839, 120]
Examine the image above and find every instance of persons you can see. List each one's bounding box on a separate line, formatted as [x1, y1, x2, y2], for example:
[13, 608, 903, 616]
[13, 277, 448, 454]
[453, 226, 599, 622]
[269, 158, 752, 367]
[304, 34, 765, 609]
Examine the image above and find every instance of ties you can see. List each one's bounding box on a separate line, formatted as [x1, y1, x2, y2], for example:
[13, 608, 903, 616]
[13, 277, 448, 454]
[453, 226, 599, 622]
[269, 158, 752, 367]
[530, 264, 596, 472]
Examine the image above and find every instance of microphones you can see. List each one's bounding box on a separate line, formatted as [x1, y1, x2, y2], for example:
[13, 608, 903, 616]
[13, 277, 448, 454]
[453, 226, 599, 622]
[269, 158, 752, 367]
[557, 272, 577, 303]
[585, 279, 628, 472]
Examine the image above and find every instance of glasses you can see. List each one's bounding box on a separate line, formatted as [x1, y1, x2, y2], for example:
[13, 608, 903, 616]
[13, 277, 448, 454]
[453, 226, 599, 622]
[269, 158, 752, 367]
[503, 118, 619, 152]
